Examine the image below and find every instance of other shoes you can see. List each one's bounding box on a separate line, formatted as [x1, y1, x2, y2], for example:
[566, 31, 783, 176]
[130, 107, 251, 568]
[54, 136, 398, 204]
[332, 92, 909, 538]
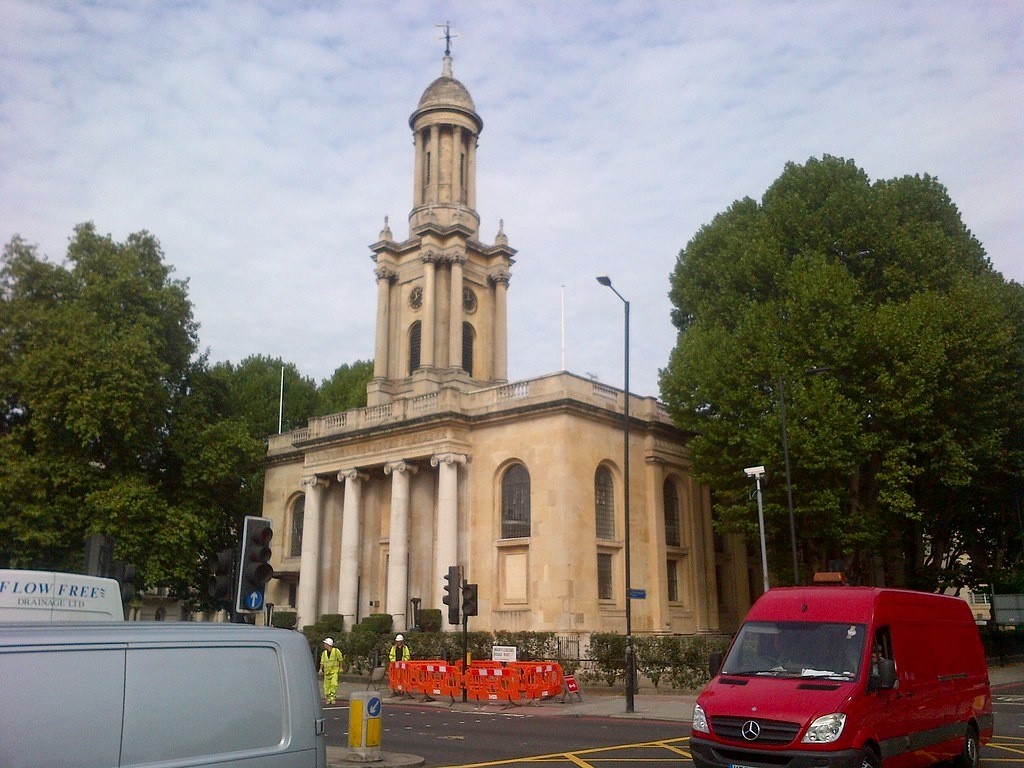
[326, 702, 330, 704]
[332, 701, 336, 704]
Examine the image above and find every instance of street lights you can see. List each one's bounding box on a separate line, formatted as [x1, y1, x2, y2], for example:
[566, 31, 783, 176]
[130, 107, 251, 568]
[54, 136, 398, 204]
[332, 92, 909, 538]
[743, 465, 769, 594]
[595, 273, 635, 713]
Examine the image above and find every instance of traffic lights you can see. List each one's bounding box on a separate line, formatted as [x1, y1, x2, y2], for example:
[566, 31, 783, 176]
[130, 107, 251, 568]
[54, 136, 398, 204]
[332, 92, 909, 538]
[442, 565, 459, 625]
[236, 516, 274, 614]
[461, 583, 479, 616]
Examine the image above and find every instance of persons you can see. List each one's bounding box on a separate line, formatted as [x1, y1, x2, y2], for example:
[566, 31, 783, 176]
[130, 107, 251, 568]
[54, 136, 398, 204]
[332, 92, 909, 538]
[860, 643, 888, 677]
[388, 634, 410, 699]
[319, 638, 343, 705]
[759, 633, 796, 661]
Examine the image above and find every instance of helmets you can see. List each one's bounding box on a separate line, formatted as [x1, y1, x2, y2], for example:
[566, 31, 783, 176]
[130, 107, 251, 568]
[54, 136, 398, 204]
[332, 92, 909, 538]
[395, 635, 404, 641]
[323, 638, 333, 646]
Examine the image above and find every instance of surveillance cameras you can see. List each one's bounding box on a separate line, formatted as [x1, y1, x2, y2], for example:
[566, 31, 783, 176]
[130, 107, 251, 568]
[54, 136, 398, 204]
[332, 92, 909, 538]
[743, 466, 766, 474]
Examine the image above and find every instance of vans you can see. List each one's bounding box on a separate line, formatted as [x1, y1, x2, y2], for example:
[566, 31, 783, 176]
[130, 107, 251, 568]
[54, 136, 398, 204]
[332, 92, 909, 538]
[0, 618, 327, 768]
[688, 572, 995, 768]
[0, 568, 124, 623]
